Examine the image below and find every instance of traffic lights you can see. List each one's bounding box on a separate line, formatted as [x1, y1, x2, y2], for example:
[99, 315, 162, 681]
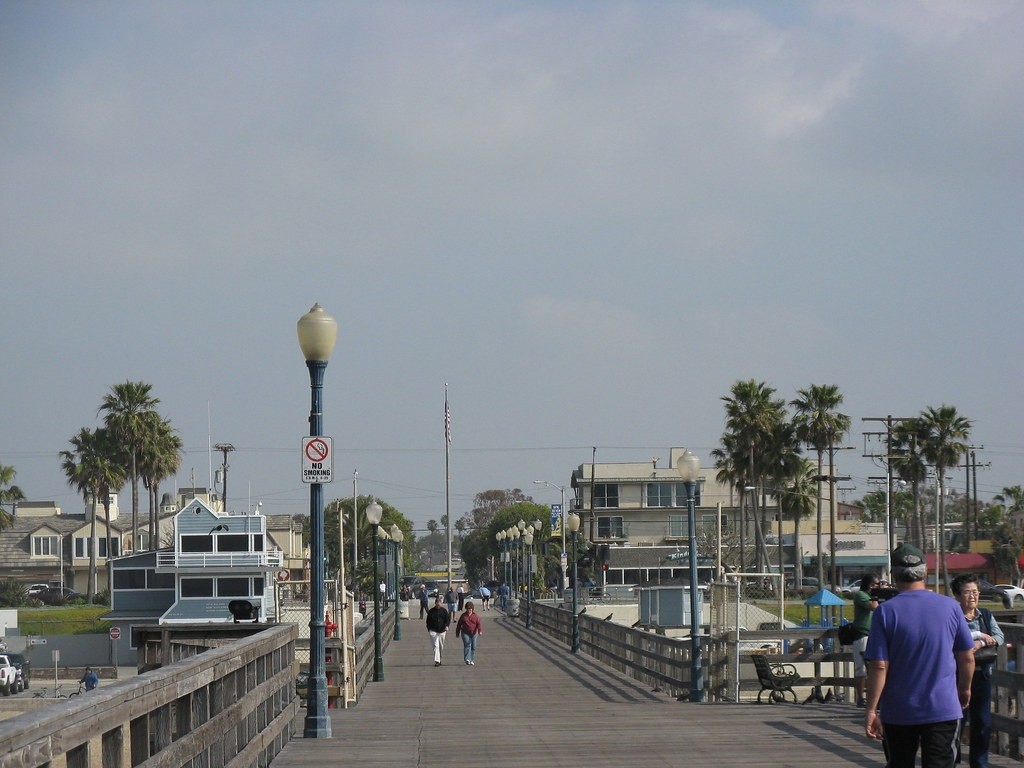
[602, 564, 609, 571]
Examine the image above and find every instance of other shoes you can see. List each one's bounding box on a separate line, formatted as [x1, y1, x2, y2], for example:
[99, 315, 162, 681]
[435, 662, 440, 667]
[454, 620, 456, 623]
[465, 659, 470, 665]
[856, 699, 868, 708]
[471, 661, 475, 665]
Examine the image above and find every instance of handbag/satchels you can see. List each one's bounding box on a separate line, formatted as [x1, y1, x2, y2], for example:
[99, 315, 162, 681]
[974, 645, 999, 665]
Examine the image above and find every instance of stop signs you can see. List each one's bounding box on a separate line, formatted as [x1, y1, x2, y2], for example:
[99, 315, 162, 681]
[110, 628, 120, 638]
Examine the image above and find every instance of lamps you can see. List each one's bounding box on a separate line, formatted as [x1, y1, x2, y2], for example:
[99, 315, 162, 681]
[213, 524, 229, 532]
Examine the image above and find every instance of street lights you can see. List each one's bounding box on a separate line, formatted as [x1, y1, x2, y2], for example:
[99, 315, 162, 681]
[744, 486, 837, 626]
[935, 477, 953, 593]
[512, 525, 520, 599]
[296, 302, 403, 739]
[518, 518, 526, 598]
[501, 527, 513, 599]
[524, 480, 565, 629]
[567, 512, 581, 654]
[677, 449, 707, 703]
[887, 474, 906, 586]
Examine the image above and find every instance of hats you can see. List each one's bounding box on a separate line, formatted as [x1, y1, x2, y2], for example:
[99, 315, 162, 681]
[420, 584, 425, 588]
[450, 586, 454, 590]
[86, 666, 92, 671]
[891, 543, 926, 567]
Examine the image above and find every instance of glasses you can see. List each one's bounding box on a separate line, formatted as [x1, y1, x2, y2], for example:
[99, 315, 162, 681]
[961, 590, 980, 597]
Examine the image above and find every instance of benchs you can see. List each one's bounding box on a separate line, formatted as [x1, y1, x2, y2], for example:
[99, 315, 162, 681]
[751, 655, 800, 704]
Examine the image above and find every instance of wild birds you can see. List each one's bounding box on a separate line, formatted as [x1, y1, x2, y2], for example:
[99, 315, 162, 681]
[824, 687, 834, 704]
[579, 607, 587, 614]
[603, 613, 613, 621]
[209, 524, 230, 536]
[631, 620, 641, 628]
[802, 688, 815, 705]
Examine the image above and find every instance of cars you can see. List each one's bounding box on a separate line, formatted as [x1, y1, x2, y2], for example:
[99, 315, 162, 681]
[755, 622, 786, 632]
[668, 627, 749, 654]
[0, 584, 92, 698]
[785, 576, 820, 588]
[977, 580, 1013, 608]
[979, 584, 1024, 606]
[401, 576, 510, 599]
[840, 579, 888, 593]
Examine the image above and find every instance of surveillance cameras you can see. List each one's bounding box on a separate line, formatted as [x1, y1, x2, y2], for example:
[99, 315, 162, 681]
[259, 502, 262, 506]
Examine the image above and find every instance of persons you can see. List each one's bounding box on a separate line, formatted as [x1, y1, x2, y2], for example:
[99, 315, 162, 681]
[77, 667, 98, 693]
[864, 542, 975, 768]
[852, 573, 895, 708]
[380, 580, 510, 619]
[455, 602, 484, 666]
[425, 597, 452, 665]
[948, 574, 1004, 768]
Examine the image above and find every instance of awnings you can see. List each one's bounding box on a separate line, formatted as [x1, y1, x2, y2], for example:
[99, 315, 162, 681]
[801, 557, 889, 567]
